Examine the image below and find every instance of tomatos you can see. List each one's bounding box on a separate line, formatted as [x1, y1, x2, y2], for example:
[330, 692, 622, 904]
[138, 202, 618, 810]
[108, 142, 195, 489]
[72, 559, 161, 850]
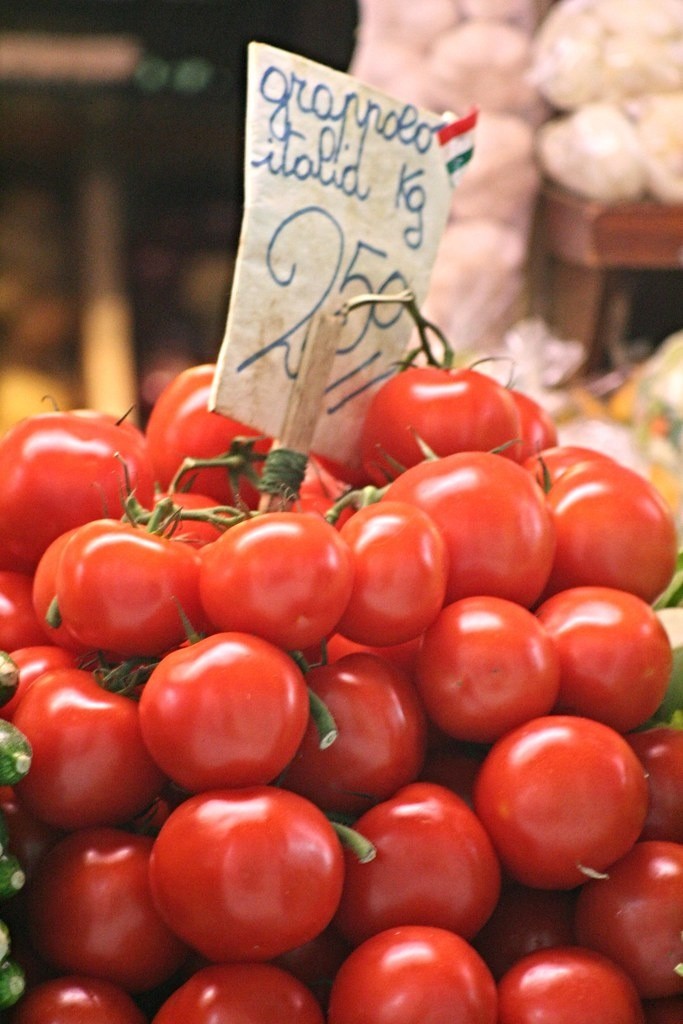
[0, 361, 683, 1024]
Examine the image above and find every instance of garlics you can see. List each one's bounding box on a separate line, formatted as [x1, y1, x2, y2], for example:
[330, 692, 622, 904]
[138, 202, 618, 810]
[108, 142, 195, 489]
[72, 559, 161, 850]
[337, 0, 683, 367]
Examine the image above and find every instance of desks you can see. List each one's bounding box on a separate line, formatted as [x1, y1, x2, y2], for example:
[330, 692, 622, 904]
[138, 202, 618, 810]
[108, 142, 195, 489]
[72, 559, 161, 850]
[534, 182, 682, 390]
